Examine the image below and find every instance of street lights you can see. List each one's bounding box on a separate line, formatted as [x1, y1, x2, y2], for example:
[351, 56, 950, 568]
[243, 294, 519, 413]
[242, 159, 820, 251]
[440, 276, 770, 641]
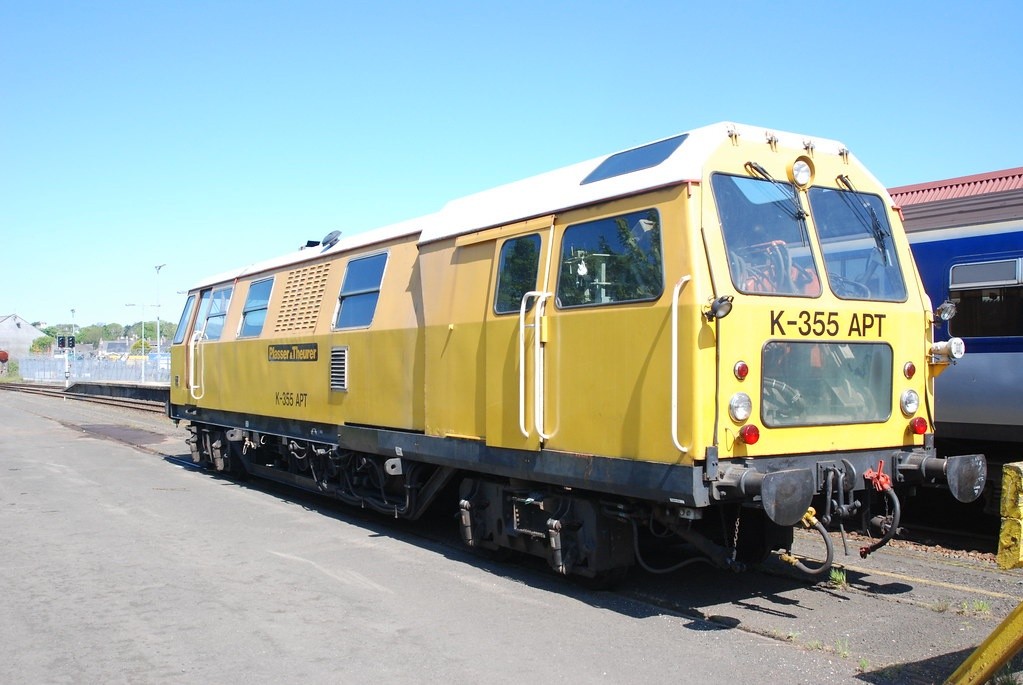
[125, 303, 161, 384]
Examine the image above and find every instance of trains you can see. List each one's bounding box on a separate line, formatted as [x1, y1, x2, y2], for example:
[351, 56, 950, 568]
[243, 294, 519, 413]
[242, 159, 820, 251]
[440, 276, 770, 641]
[165, 120, 987, 590]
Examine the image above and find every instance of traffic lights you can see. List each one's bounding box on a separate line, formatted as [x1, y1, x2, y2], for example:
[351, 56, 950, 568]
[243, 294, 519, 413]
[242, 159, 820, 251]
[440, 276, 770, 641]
[58, 336, 65, 347]
[68, 336, 75, 347]
